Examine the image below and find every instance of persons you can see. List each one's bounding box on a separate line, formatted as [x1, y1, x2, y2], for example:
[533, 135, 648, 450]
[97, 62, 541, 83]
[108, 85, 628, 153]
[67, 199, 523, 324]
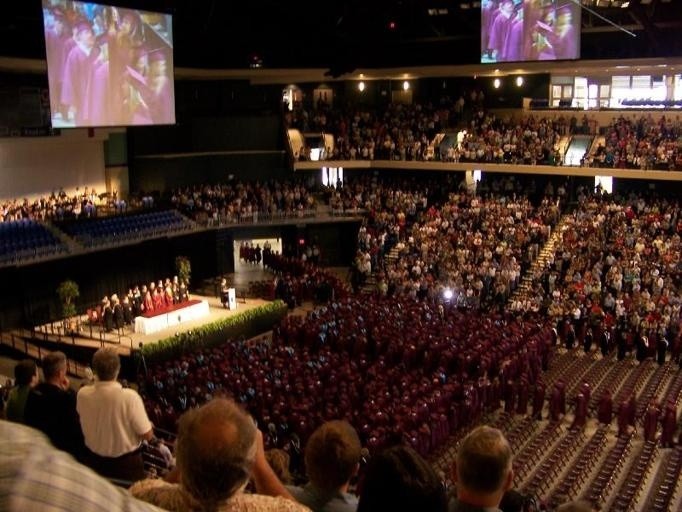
[40, 1, 177, 129]
[482, 0, 582, 63]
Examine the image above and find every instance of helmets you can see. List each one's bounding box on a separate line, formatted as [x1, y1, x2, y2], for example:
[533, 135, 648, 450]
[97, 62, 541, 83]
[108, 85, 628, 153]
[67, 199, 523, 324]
[121, 65, 148, 93]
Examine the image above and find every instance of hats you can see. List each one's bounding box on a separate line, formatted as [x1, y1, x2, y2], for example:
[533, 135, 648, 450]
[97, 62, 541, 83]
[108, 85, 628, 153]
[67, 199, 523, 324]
[541, 5, 555, 16]
[555, 3, 572, 18]
[74, 22, 92, 42]
[147, 48, 165, 62]
[534, 20, 553, 38]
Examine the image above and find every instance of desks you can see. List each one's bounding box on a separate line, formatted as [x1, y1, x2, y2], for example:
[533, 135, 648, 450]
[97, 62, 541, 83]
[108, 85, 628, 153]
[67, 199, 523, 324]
[134, 299, 210, 334]
